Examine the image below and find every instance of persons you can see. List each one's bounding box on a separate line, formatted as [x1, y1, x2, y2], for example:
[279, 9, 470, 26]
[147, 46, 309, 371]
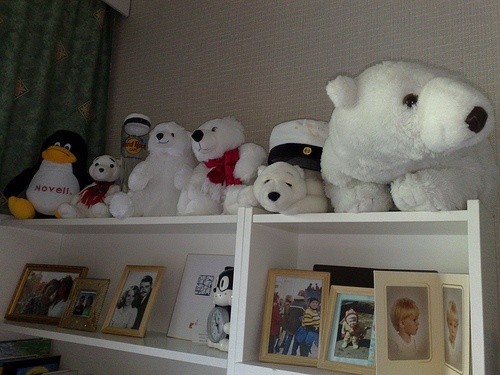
[74, 294, 95, 316]
[444, 299, 462, 366]
[342, 309, 367, 350]
[268, 283, 321, 356]
[21, 274, 74, 318]
[109, 274, 153, 331]
[389, 296, 421, 359]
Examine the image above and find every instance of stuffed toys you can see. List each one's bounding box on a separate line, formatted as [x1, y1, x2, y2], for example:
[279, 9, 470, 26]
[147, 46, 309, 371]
[321, 61, 497, 213]
[3, 118, 330, 221]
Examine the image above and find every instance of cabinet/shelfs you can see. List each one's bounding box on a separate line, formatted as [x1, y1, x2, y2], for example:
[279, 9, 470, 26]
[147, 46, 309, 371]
[0, 193, 490, 375]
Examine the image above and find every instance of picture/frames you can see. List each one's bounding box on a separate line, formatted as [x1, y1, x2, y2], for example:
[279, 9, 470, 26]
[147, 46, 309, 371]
[4, 264, 89, 326]
[440, 272, 469, 375]
[317, 285, 375, 375]
[58, 278, 111, 331]
[374, 270, 440, 375]
[100, 264, 166, 338]
[258, 269, 331, 367]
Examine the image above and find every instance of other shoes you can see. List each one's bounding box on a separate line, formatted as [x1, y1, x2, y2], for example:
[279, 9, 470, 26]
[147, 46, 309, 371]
[341, 340, 350, 348]
[352, 342, 358, 349]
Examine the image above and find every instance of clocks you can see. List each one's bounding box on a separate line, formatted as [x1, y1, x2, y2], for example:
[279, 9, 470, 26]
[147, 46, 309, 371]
[206, 266, 234, 351]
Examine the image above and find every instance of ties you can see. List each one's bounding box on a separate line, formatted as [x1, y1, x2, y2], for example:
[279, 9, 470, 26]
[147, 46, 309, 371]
[140, 296, 144, 304]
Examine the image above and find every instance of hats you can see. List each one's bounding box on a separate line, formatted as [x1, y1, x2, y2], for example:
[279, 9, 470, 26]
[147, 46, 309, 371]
[309, 297, 319, 303]
